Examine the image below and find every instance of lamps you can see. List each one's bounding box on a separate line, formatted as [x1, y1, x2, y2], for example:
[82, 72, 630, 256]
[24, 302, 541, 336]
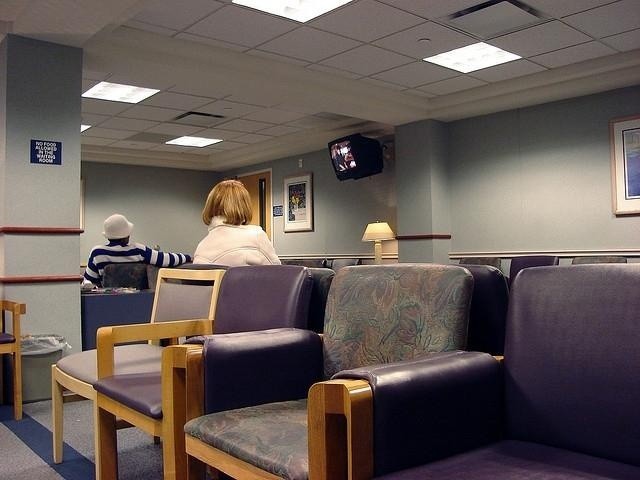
[361, 218, 397, 266]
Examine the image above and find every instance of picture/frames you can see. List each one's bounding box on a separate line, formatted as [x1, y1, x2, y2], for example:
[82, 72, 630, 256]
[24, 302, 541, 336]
[607, 115, 640, 215]
[281, 171, 314, 233]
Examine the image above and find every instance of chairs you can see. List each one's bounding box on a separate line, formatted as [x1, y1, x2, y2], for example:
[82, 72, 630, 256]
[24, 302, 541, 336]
[282, 260, 301, 265]
[461, 256, 501, 268]
[461, 266, 508, 357]
[50, 267, 227, 461]
[0, 297, 27, 421]
[101, 264, 148, 290]
[300, 259, 326, 267]
[182, 260, 475, 480]
[570, 257, 630, 266]
[308, 266, 336, 335]
[508, 256, 560, 287]
[332, 258, 359, 273]
[147, 265, 177, 289]
[342, 263, 640, 480]
[91, 267, 296, 479]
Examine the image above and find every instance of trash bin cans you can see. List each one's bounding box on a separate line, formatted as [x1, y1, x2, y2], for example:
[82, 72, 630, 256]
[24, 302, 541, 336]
[20, 333, 68, 404]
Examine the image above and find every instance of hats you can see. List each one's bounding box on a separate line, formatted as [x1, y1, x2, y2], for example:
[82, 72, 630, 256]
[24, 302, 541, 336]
[101, 214, 135, 240]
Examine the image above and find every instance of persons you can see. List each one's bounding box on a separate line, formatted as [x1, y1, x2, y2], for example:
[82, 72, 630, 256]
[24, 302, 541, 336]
[81, 214, 193, 289]
[193, 180, 281, 266]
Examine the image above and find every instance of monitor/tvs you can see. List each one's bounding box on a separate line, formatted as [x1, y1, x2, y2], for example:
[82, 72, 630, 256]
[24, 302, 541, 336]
[328, 133, 384, 182]
